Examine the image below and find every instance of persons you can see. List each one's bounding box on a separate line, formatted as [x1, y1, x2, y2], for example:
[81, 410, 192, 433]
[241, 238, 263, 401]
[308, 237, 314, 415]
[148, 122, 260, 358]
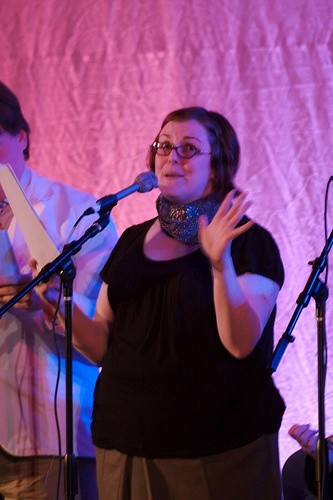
[0, 82, 119, 500]
[288, 424, 333, 466]
[0, 107, 286, 500]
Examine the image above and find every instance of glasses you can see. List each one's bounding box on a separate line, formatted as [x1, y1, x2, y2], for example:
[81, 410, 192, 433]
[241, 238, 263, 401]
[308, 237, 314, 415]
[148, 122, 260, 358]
[150, 141, 213, 159]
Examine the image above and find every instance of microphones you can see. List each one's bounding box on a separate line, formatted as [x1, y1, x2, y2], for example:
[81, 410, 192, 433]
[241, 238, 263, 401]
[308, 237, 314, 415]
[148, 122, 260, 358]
[85, 171, 158, 215]
